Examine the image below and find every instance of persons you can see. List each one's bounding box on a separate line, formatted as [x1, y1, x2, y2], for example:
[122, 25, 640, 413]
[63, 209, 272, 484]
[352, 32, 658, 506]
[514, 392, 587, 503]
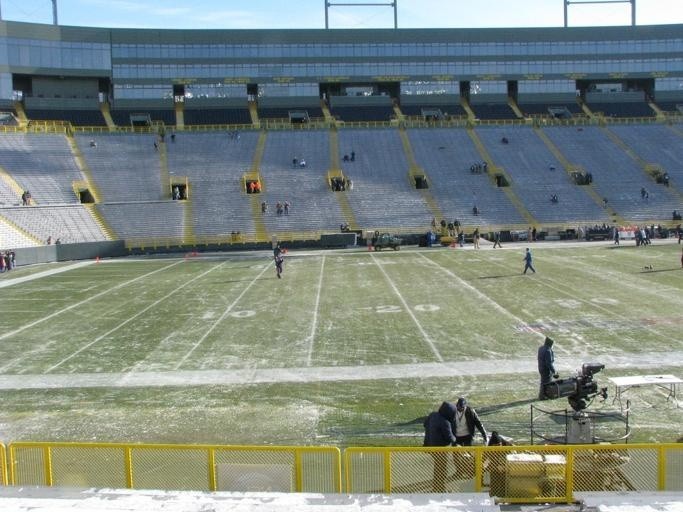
[275, 252, 284, 279]
[340, 222, 351, 231]
[0, 248, 15, 273]
[261, 200, 265, 214]
[173, 184, 186, 200]
[292, 156, 298, 167]
[248, 181, 255, 193]
[253, 182, 260, 193]
[602, 197, 607, 209]
[284, 200, 289, 214]
[641, 186, 646, 200]
[343, 154, 349, 162]
[55, 237, 60, 244]
[570, 170, 592, 185]
[471, 205, 478, 215]
[329, 175, 353, 193]
[299, 158, 305, 167]
[24, 190, 31, 205]
[276, 201, 283, 215]
[421, 401, 465, 493]
[519, 248, 535, 274]
[46, 236, 51, 245]
[487, 431, 514, 460]
[469, 160, 489, 174]
[537, 335, 559, 400]
[350, 150, 355, 161]
[647, 168, 671, 188]
[21, 191, 27, 206]
[273, 244, 282, 262]
[550, 194, 558, 203]
[452, 397, 489, 480]
[430, 209, 682, 249]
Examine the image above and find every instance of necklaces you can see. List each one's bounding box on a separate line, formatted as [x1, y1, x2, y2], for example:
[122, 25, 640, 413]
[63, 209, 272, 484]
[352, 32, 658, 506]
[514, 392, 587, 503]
[456, 411, 463, 424]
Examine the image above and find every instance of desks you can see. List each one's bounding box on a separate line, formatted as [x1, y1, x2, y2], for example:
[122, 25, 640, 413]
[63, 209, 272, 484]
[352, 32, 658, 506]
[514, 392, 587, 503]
[609, 374, 683, 412]
[618, 230, 646, 240]
[321, 233, 357, 248]
